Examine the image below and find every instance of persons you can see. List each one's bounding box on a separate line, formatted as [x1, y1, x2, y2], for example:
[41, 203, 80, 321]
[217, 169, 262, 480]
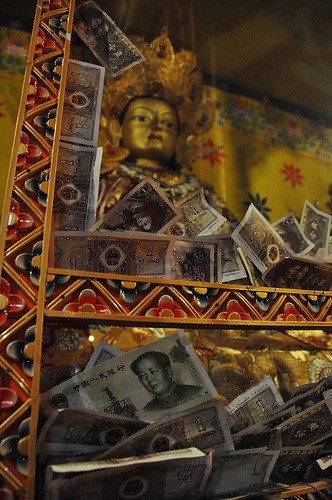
[92, 93, 244, 238]
[131, 352, 203, 411]
